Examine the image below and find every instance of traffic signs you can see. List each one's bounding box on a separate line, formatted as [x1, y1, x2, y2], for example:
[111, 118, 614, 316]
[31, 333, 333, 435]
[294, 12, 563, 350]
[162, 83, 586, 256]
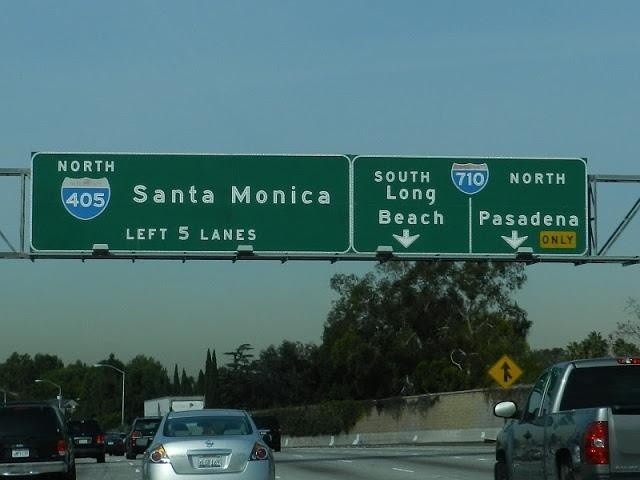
[29, 151, 351, 253]
[353, 156, 590, 260]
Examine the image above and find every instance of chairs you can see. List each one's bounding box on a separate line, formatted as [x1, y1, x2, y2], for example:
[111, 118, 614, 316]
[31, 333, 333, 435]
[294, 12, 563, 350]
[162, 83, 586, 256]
[171, 422, 190, 435]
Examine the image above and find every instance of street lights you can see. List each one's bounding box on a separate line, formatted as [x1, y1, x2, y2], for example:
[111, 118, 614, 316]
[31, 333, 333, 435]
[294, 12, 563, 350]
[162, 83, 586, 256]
[93, 363, 128, 424]
[34, 378, 63, 407]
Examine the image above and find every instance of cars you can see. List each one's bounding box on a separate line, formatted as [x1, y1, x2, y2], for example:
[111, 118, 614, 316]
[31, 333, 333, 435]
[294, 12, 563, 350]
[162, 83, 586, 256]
[126, 416, 163, 457]
[104, 431, 126, 458]
[2, 403, 105, 479]
[144, 409, 275, 480]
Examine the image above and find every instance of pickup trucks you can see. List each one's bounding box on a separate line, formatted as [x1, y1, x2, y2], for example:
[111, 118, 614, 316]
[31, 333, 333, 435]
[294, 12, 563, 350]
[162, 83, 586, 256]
[493, 356, 640, 480]
